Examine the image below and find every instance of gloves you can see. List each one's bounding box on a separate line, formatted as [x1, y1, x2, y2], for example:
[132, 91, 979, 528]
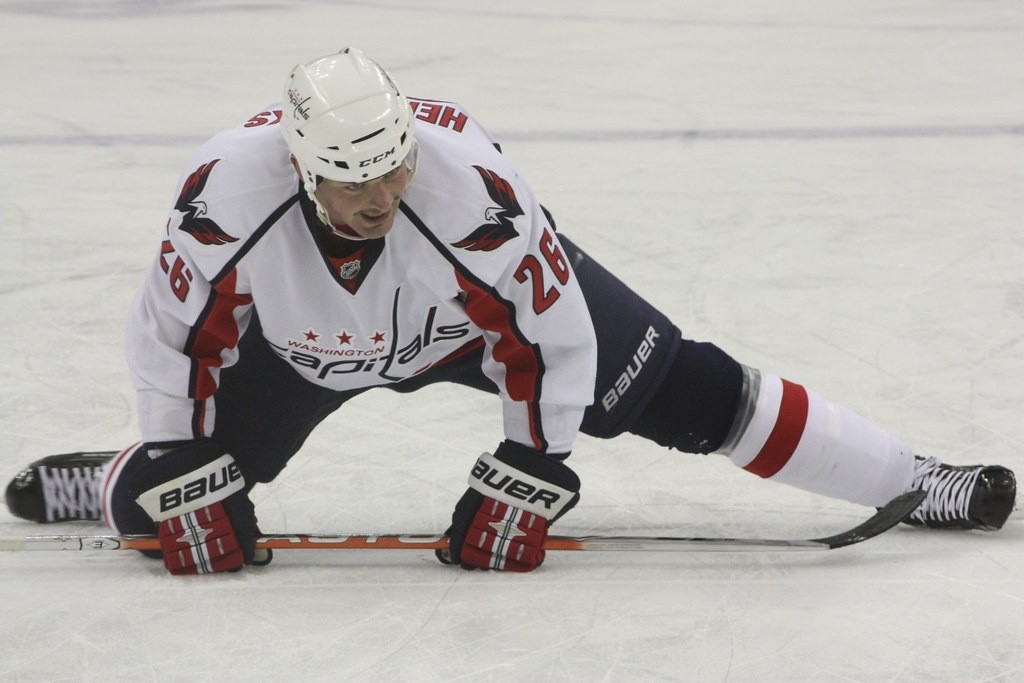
[435, 439, 581, 573]
[129, 439, 272, 575]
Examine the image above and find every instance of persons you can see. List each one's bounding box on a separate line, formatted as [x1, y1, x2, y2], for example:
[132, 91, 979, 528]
[0, 47, 1024, 575]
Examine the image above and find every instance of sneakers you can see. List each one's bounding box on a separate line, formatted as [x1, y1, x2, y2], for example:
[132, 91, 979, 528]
[876, 454, 1021, 532]
[6, 449, 120, 524]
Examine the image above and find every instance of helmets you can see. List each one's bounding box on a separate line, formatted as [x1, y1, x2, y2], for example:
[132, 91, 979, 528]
[282, 47, 419, 191]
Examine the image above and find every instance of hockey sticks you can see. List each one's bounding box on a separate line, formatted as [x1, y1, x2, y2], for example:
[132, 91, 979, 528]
[0, 484, 927, 564]
